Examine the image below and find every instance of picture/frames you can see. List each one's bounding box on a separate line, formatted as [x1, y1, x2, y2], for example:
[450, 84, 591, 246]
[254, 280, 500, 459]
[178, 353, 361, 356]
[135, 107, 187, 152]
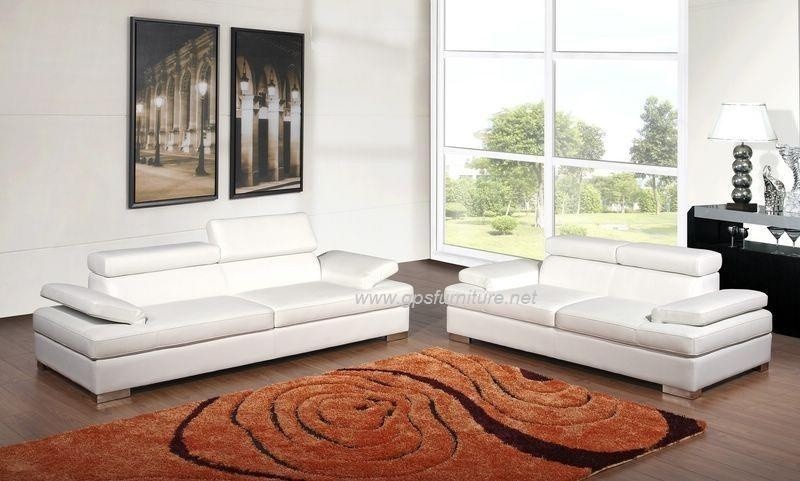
[127, 17, 305, 209]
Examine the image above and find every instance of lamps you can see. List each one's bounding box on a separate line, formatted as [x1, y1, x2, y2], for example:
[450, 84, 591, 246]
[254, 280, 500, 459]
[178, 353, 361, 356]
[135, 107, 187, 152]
[707, 102, 778, 213]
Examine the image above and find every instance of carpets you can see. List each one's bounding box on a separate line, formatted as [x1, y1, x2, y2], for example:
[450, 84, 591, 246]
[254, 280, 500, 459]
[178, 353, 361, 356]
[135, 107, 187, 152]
[0, 342, 708, 480]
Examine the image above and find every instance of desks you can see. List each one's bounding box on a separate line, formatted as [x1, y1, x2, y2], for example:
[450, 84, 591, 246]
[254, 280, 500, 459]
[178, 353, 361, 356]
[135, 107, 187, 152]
[687, 204, 800, 339]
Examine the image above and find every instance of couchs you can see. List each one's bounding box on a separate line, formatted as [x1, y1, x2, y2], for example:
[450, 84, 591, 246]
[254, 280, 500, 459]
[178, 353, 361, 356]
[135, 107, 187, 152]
[442, 234, 774, 403]
[31, 213, 416, 406]
